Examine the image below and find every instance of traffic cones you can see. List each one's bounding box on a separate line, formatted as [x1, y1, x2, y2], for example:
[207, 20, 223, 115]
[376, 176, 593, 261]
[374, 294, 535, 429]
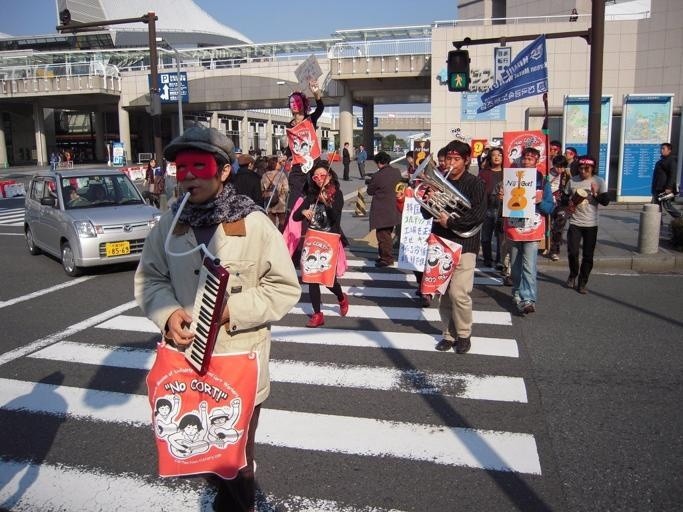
[352, 186, 367, 217]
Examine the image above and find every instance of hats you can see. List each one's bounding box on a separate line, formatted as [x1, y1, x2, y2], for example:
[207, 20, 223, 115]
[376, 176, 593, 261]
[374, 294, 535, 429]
[239, 154, 254, 165]
[163, 127, 236, 163]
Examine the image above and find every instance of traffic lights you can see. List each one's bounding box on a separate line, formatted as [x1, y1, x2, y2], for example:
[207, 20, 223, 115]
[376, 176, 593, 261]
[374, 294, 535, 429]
[447, 50, 469, 91]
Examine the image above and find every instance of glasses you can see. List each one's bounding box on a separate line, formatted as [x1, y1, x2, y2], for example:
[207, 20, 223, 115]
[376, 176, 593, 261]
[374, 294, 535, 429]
[312, 175, 327, 182]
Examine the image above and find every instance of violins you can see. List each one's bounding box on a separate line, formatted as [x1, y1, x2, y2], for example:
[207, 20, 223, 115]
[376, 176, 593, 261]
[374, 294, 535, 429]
[304, 149, 343, 225]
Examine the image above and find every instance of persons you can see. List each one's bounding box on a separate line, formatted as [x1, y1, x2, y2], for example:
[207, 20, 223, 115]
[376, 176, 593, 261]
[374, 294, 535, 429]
[650, 141, 682, 234]
[49, 148, 71, 171]
[62, 185, 83, 207]
[90, 183, 106, 199]
[129, 123, 301, 512]
[145, 140, 614, 330]
[283, 79, 327, 217]
[417, 138, 488, 355]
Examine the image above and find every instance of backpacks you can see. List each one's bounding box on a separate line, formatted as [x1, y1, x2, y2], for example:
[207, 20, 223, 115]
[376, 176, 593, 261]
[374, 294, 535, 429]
[262, 172, 279, 208]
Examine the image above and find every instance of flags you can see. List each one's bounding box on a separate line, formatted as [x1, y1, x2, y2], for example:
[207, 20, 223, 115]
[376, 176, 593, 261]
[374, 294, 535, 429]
[474, 34, 551, 113]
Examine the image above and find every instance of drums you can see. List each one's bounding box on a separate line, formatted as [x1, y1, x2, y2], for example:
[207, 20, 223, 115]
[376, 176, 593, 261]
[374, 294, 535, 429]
[560, 189, 588, 224]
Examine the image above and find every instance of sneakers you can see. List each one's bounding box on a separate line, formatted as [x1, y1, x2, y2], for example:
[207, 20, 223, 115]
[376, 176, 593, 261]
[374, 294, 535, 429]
[306, 251, 588, 354]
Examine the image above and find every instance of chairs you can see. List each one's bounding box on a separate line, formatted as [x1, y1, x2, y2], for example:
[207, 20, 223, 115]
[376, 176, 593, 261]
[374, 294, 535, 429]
[86, 184, 104, 201]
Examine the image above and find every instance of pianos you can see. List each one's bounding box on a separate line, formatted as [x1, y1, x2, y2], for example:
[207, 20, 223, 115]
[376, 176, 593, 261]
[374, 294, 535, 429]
[181, 256, 230, 376]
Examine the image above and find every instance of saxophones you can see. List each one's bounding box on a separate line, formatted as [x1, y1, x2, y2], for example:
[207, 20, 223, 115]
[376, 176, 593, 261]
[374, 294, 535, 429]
[406, 154, 485, 239]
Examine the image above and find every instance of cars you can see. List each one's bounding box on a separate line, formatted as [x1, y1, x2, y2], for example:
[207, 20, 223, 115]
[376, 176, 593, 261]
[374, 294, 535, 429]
[21, 168, 163, 274]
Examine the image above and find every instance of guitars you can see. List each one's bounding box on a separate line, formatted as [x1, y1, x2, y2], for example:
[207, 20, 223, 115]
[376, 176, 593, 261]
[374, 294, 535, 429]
[155, 419, 177, 441]
[507, 170, 530, 212]
[167, 437, 216, 460]
[206, 427, 244, 450]
